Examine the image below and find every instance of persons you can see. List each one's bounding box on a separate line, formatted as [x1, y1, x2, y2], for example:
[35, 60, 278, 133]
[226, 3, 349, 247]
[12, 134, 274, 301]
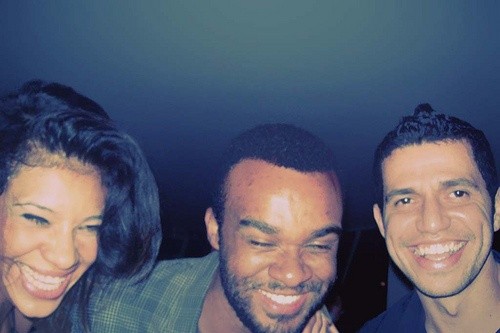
[2, 80, 163, 333]
[354, 103, 500, 333]
[70, 123, 349, 333]
